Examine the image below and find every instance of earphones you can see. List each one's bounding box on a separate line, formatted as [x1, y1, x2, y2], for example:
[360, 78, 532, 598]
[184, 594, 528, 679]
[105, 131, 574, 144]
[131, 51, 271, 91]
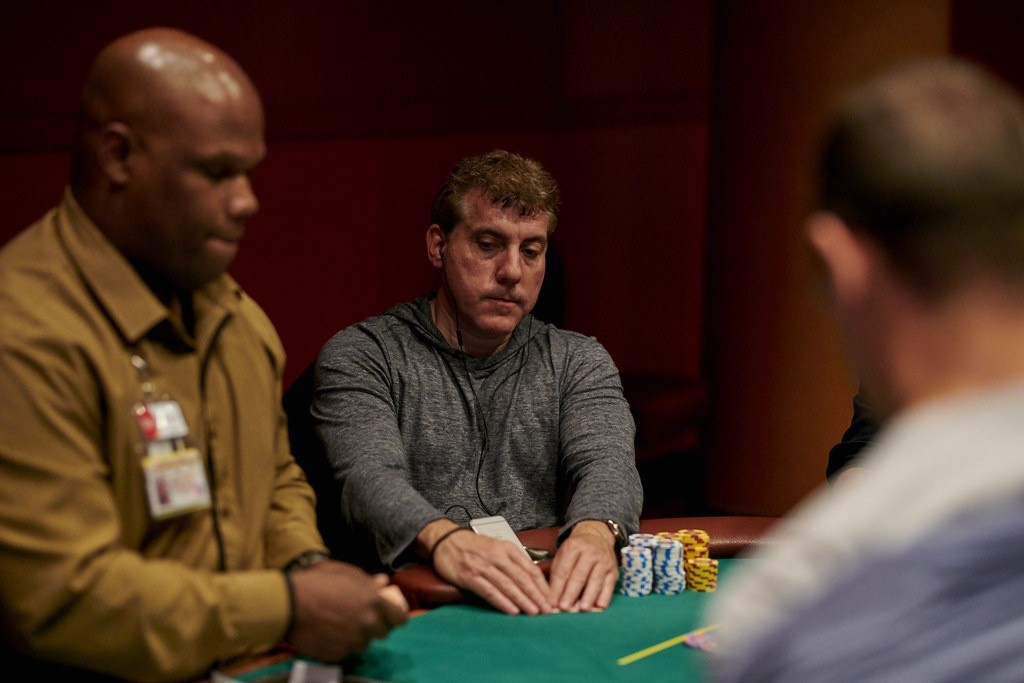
[439, 248, 446, 261]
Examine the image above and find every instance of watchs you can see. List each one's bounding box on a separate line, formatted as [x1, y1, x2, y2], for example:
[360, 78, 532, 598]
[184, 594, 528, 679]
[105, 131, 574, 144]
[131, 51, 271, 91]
[606, 519, 625, 545]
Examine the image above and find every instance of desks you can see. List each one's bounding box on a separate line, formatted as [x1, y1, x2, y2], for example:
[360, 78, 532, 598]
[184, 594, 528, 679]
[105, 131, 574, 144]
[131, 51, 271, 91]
[204, 516, 784, 683]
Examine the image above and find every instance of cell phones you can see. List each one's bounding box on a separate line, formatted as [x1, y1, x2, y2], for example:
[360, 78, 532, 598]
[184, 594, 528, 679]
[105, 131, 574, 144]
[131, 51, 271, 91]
[470, 515, 533, 564]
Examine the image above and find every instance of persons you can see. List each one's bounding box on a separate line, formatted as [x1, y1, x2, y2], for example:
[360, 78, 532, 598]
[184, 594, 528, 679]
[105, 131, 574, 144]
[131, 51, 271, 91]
[0, 27, 407, 683]
[311, 149, 644, 615]
[708, 58, 1024, 683]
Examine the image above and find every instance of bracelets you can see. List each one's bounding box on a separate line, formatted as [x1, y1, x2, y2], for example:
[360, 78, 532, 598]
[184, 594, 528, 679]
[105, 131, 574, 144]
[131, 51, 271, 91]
[429, 526, 473, 562]
